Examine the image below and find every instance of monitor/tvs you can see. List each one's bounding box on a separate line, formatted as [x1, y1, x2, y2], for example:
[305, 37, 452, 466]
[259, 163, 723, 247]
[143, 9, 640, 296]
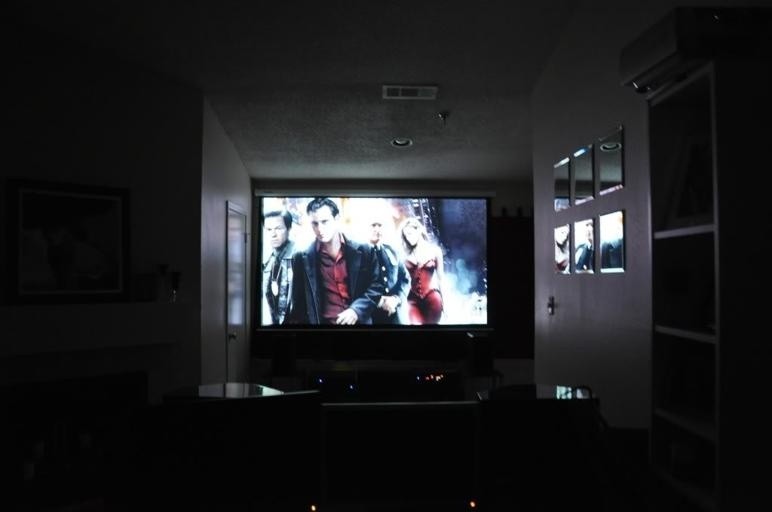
[253, 189, 498, 340]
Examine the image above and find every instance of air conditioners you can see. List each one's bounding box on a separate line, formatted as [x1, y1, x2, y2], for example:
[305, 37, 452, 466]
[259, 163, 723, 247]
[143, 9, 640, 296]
[618, 6, 758, 93]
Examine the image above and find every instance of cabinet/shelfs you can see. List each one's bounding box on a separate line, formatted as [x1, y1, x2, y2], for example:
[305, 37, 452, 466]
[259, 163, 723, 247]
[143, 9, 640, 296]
[644, 59, 769, 509]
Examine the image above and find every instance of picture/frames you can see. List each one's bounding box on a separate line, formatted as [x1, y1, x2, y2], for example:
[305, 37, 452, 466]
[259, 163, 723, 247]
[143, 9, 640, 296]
[3, 182, 131, 307]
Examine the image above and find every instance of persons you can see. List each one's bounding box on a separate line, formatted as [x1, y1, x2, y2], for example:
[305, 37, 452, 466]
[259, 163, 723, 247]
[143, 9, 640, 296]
[357, 221, 409, 325]
[574, 223, 594, 271]
[402, 217, 448, 324]
[555, 233, 570, 275]
[263, 209, 297, 325]
[292, 199, 385, 326]
